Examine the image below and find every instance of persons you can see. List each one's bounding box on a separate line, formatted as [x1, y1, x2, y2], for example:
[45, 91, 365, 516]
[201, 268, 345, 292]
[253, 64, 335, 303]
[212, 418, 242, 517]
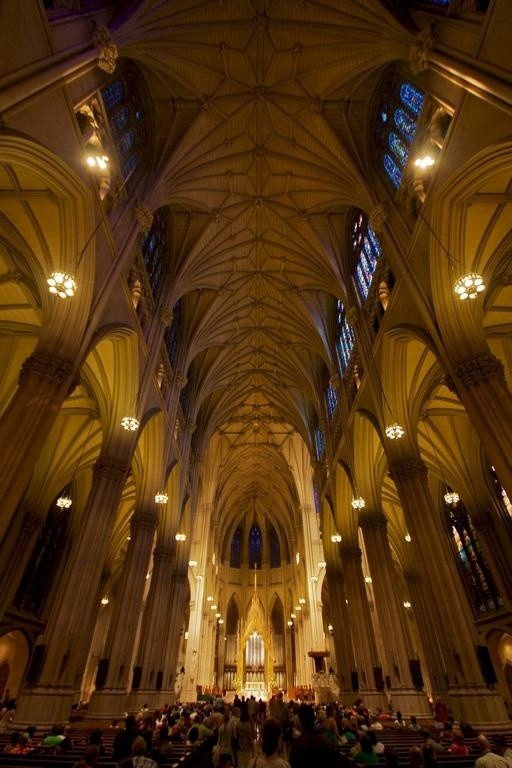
[0, 688, 512, 768]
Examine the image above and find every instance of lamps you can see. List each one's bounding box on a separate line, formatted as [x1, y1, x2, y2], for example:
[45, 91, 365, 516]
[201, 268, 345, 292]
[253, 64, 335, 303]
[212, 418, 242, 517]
[44, 111, 172, 299]
[155, 491, 169, 507]
[174, 530, 187, 543]
[349, 492, 367, 513]
[314, 514, 329, 570]
[404, 185, 485, 301]
[329, 527, 343, 544]
[117, 394, 141, 433]
[186, 495, 201, 569]
[85, 113, 146, 172]
[54, 417, 95, 511]
[326, 230, 406, 442]
[100, 572, 420, 628]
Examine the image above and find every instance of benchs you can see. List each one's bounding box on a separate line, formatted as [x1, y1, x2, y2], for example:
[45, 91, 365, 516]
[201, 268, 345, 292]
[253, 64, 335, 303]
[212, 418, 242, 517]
[0, 716, 213, 768]
[322, 721, 512, 768]
